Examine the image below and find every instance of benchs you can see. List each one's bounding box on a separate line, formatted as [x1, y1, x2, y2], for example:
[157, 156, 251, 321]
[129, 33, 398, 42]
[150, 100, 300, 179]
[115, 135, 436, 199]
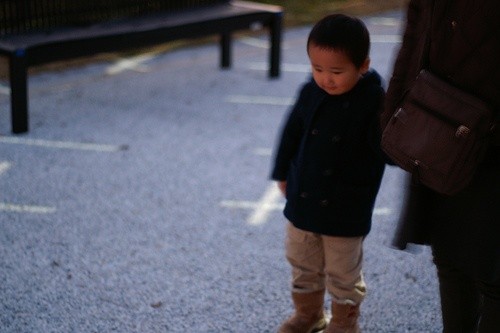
[0, 0, 283, 134]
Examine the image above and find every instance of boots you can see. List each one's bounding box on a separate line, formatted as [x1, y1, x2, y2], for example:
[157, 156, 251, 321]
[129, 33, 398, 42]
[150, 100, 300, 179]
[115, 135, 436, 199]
[277, 284, 325, 333]
[318, 300, 362, 333]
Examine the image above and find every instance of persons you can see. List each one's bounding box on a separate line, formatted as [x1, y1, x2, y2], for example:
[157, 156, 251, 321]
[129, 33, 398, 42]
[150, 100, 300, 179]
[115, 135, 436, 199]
[271, 13, 390, 332]
[382, 0, 500, 333]
